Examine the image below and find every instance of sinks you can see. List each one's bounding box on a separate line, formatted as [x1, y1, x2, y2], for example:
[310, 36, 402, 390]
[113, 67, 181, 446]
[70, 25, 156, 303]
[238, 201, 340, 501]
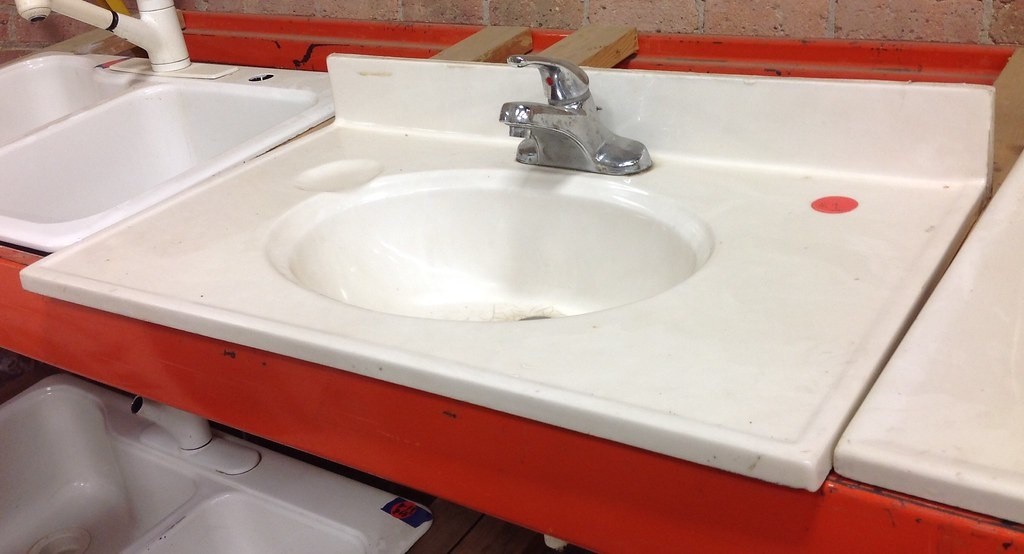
[0, 53, 156, 154]
[1, 372, 232, 554]
[261, 166, 715, 325]
[129, 446, 436, 553]
[0, 79, 320, 255]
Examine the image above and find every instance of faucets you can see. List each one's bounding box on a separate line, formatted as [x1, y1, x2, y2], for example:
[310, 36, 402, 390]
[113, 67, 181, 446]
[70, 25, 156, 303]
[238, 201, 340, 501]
[497, 55, 653, 175]
[13, 0, 191, 73]
